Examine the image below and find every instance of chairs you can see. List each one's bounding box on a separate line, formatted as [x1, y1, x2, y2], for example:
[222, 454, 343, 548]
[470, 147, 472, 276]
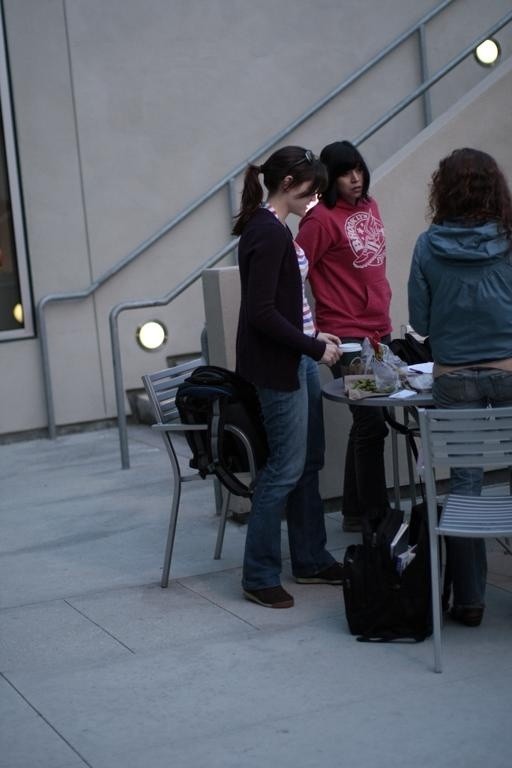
[139, 356, 269, 590]
[415, 406, 511, 676]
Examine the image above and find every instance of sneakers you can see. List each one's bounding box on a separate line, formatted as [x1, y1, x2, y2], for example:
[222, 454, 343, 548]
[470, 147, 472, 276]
[340, 518, 365, 534]
[294, 563, 346, 586]
[242, 581, 295, 608]
[448, 603, 483, 627]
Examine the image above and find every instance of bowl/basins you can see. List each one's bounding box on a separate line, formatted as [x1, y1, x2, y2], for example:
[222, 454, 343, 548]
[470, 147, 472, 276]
[401, 362, 434, 393]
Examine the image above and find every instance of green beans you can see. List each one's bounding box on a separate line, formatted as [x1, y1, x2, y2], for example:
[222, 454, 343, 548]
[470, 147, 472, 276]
[352, 378, 395, 394]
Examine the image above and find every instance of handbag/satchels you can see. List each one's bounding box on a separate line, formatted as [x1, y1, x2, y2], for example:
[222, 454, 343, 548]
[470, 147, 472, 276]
[342, 509, 434, 643]
[407, 501, 455, 614]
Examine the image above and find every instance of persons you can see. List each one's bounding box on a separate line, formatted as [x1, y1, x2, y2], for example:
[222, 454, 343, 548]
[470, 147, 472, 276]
[407, 143, 512, 627]
[229, 143, 350, 609]
[295, 140, 402, 535]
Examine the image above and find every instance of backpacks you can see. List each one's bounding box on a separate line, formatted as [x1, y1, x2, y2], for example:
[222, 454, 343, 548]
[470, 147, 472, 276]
[176, 364, 266, 499]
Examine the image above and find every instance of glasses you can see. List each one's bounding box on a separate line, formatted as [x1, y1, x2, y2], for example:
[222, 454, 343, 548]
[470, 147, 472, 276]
[292, 149, 314, 173]
